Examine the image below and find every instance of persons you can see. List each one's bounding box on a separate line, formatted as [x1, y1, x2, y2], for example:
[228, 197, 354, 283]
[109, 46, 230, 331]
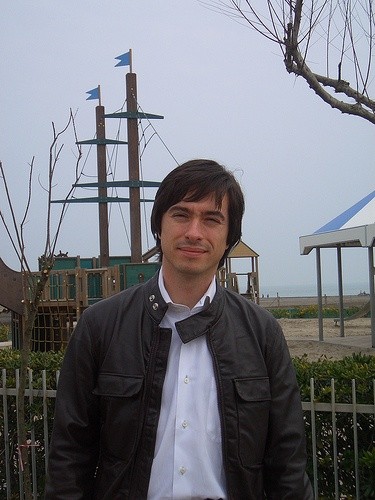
[44, 159, 317, 500]
